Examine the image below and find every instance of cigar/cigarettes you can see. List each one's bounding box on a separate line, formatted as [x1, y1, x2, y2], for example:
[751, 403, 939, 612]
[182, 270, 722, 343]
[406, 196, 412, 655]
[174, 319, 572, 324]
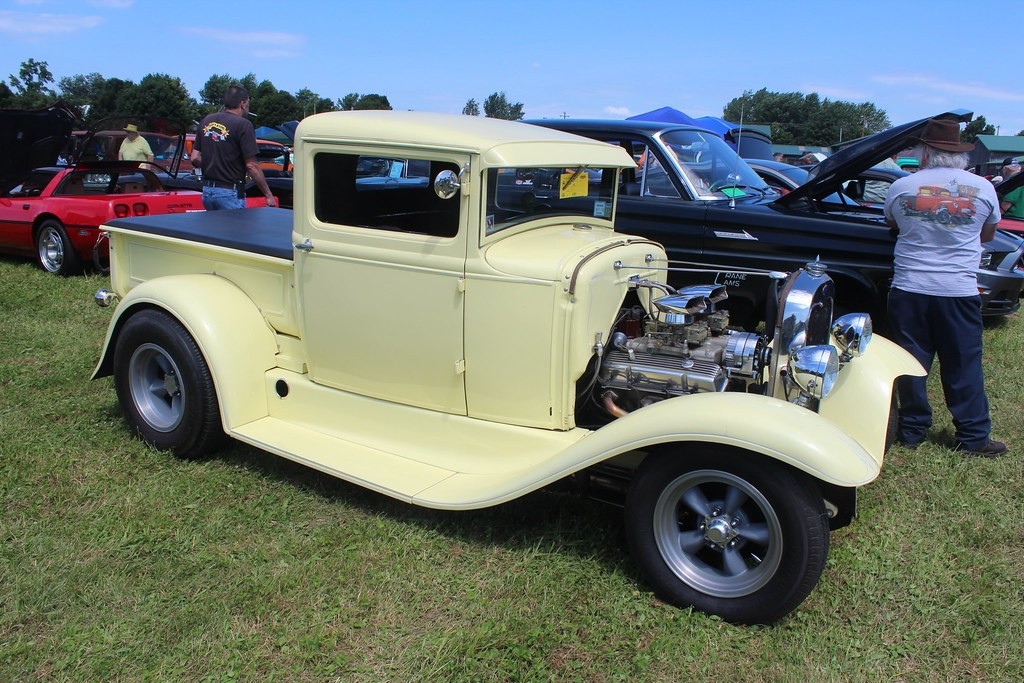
[248, 112, 257, 116]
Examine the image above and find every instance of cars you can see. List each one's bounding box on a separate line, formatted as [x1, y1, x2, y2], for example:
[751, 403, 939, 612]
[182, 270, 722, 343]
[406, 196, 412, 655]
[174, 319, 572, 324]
[85, 110, 931, 626]
[13, 118, 299, 209]
[496, 113, 1024, 334]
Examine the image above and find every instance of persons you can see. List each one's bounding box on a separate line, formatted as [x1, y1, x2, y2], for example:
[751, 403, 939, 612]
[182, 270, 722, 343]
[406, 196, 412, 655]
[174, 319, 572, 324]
[991, 173, 1024, 265]
[794, 151, 813, 166]
[635, 136, 678, 170]
[862, 154, 902, 202]
[190, 86, 276, 211]
[118, 124, 153, 170]
[883, 119, 1006, 456]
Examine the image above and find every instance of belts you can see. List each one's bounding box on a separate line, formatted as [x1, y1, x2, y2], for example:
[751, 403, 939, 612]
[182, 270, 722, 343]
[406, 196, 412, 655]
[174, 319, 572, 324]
[201, 181, 243, 190]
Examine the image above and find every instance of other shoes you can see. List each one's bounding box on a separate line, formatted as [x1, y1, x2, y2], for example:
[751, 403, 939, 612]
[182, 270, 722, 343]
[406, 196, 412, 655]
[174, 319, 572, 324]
[900, 441, 923, 451]
[953, 438, 1007, 458]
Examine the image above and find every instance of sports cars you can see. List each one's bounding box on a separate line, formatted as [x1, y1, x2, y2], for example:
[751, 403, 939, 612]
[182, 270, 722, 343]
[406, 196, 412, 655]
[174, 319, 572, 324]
[356, 154, 430, 193]
[0, 98, 280, 278]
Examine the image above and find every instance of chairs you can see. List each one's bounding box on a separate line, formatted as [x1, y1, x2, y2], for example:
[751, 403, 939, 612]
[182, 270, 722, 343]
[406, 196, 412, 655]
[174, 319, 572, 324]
[62, 179, 84, 196]
[123, 182, 143, 194]
[620, 184, 642, 196]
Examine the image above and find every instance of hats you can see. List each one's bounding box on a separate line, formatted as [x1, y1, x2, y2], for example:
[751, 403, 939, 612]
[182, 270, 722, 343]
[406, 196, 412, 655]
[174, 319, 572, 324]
[1000, 158, 1019, 171]
[904, 119, 976, 152]
[123, 124, 139, 133]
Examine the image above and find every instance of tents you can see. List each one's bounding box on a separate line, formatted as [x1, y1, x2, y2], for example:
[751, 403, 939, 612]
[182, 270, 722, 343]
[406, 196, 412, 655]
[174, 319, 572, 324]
[625, 106, 740, 151]
